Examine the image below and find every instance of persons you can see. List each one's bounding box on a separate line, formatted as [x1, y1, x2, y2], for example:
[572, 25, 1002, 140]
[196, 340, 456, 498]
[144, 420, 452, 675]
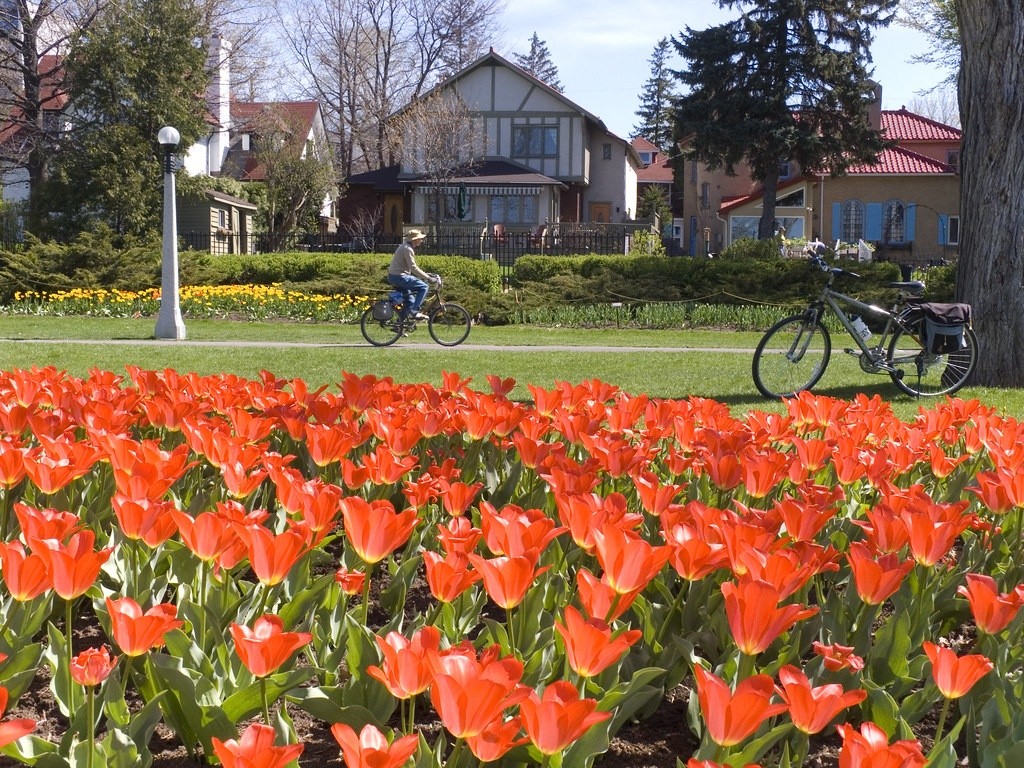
[775, 225, 787, 255]
[389, 230, 439, 337]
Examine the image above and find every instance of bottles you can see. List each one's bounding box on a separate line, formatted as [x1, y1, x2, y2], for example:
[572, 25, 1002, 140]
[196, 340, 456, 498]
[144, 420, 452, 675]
[850, 314, 872, 341]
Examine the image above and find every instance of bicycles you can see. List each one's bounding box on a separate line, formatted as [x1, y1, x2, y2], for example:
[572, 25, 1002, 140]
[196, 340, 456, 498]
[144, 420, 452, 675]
[360, 273, 472, 347]
[751, 248, 979, 402]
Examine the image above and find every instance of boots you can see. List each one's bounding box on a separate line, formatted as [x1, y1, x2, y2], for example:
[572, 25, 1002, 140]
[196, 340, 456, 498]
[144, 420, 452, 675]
[391, 328, 408, 337]
[408, 311, 428, 320]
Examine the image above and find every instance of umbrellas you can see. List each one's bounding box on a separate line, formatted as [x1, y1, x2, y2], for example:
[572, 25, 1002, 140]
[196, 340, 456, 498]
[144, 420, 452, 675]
[457, 180, 466, 221]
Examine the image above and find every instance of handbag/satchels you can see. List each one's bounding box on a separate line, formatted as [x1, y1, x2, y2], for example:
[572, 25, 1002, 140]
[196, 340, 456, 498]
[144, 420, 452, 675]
[373, 301, 393, 320]
[919, 300, 971, 353]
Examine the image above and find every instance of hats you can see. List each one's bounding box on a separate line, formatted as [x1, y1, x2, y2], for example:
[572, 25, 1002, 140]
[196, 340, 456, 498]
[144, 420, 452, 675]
[404, 229, 427, 242]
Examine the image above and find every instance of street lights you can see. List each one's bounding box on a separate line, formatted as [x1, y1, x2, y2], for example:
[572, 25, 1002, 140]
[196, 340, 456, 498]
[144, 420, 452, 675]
[152, 124, 187, 342]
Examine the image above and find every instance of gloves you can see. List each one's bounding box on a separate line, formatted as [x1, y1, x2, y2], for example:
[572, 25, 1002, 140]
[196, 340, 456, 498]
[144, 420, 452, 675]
[428, 277, 437, 284]
